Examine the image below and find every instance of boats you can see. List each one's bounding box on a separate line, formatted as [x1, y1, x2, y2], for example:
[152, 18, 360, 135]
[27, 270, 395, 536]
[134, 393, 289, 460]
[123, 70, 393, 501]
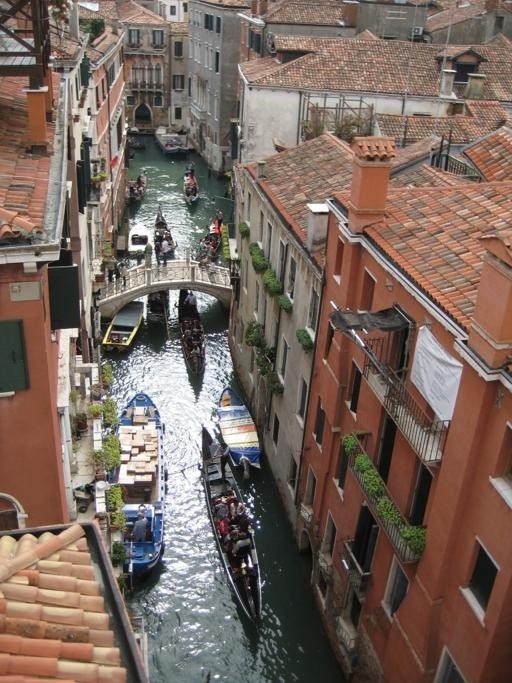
[153, 204, 175, 261]
[182, 170, 199, 205]
[103, 299, 144, 352]
[188, 230, 223, 264]
[128, 221, 150, 257]
[128, 172, 147, 200]
[127, 135, 146, 150]
[154, 126, 188, 155]
[146, 287, 169, 334]
[200, 422, 266, 630]
[176, 285, 207, 375]
[105, 392, 165, 578]
[211, 387, 262, 484]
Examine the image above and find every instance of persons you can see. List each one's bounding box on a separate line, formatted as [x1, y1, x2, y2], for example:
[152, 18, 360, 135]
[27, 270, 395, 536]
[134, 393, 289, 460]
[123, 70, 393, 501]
[215, 493, 251, 552]
[156, 228, 174, 255]
[210, 442, 230, 480]
[185, 171, 197, 196]
[192, 208, 223, 260]
[131, 513, 148, 541]
[181, 319, 203, 354]
[107, 256, 128, 286]
[132, 175, 145, 195]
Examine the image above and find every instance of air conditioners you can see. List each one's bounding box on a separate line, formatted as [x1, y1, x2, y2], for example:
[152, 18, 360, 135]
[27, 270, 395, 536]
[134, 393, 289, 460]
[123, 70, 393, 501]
[410, 26, 424, 36]
